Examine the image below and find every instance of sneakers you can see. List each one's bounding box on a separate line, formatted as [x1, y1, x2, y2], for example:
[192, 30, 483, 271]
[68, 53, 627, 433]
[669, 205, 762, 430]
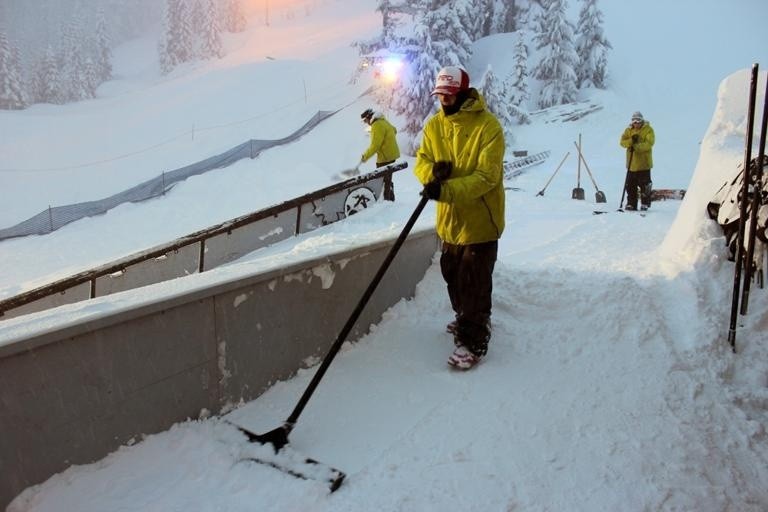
[625, 204, 648, 211]
[446, 320, 480, 369]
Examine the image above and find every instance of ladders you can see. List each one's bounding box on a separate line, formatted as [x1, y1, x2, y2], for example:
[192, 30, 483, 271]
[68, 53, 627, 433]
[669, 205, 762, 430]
[503, 149, 552, 180]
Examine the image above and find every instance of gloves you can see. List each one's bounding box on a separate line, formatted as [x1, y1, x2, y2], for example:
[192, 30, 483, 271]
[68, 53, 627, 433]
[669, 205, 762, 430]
[420, 182, 439, 201]
[433, 160, 451, 180]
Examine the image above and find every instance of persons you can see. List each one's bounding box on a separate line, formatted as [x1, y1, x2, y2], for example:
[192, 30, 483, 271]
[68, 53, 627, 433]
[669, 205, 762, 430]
[619, 111, 657, 212]
[412, 68, 507, 371]
[359, 109, 400, 203]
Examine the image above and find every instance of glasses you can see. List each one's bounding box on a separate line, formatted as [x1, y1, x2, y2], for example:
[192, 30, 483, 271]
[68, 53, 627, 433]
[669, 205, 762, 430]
[631, 117, 643, 123]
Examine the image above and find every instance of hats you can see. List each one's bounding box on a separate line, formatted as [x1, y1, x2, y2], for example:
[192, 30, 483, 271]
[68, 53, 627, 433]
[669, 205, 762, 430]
[429, 66, 469, 96]
[631, 111, 643, 124]
[361, 109, 373, 120]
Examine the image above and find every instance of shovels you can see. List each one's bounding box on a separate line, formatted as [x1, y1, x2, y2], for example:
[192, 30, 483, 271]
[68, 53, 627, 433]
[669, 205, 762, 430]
[214, 178, 435, 504]
[330, 158, 364, 183]
[536, 151, 569, 197]
[572, 133, 607, 203]
